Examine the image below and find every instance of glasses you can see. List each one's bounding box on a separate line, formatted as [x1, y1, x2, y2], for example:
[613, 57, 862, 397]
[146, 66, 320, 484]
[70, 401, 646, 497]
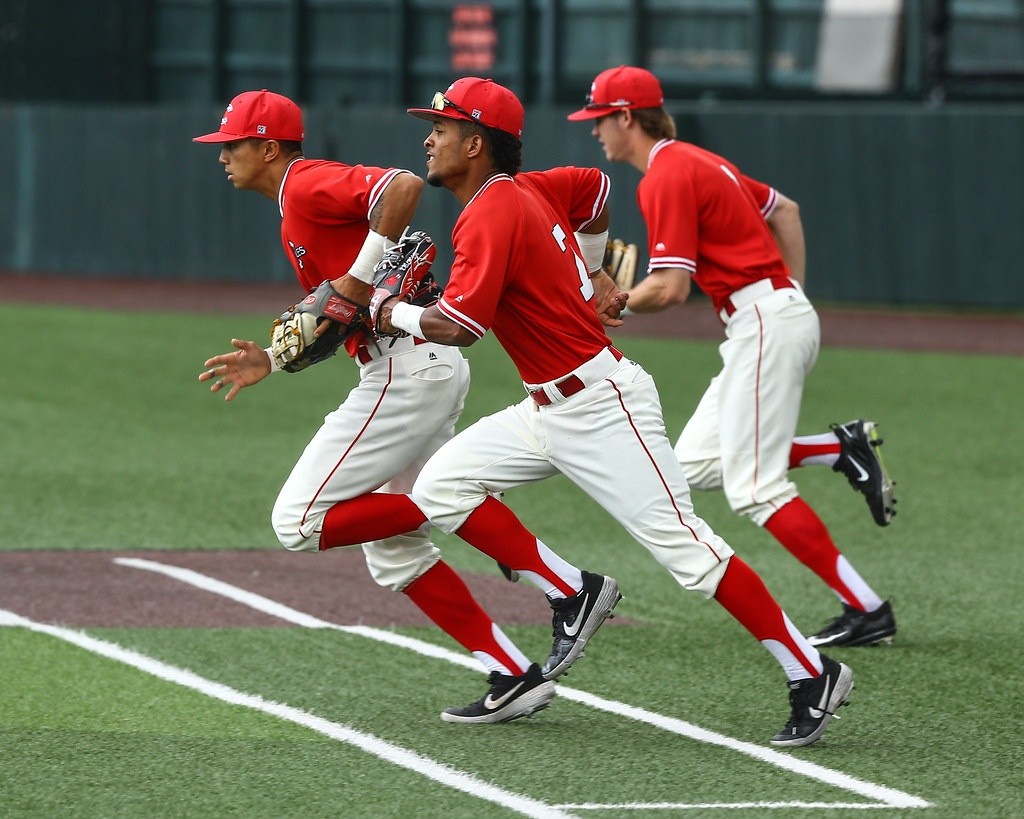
[431, 91, 489, 130]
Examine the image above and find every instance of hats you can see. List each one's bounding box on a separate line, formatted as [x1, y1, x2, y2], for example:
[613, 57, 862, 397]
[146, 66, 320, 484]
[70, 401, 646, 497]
[566, 65, 664, 122]
[407, 77, 525, 139]
[192, 88, 304, 143]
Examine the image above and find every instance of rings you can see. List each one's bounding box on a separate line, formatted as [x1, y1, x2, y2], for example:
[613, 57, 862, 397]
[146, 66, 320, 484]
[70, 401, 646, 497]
[216, 380, 223, 389]
[208, 369, 215, 379]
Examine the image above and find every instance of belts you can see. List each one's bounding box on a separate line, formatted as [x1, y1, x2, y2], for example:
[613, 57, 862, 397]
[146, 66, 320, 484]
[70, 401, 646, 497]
[530, 344, 624, 406]
[358, 336, 428, 364]
[719, 277, 796, 326]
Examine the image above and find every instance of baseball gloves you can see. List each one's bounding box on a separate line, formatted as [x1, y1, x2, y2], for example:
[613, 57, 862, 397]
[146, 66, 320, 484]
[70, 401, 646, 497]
[370, 232, 443, 341]
[271, 282, 370, 375]
[601, 237, 640, 292]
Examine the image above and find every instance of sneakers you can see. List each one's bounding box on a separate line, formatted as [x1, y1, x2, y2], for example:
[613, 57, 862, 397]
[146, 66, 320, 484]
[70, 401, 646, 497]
[541, 571, 622, 683]
[806, 595, 897, 648]
[769, 653, 854, 746]
[829, 418, 897, 527]
[440, 662, 555, 723]
[487, 491, 520, 582]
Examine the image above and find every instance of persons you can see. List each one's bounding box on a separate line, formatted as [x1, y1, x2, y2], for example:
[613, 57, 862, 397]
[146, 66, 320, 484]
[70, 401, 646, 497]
[190, 88, 623, 723]
[565, 63, 899, 648]
[370, 77, 856, 748]
